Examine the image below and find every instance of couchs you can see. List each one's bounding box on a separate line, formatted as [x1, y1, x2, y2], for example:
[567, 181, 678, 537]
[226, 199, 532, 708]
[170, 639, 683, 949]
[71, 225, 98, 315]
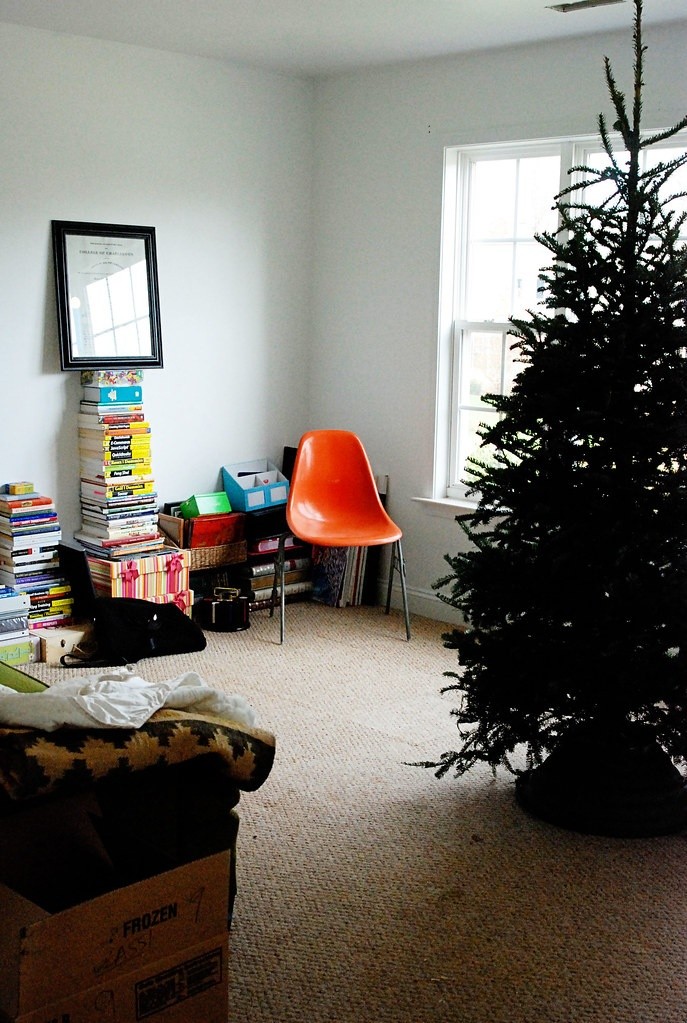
[0, 662, 276, 931]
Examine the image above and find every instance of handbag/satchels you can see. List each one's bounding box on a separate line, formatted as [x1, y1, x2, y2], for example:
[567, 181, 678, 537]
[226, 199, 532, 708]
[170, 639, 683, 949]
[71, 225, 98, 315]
[59, 597, 210, 668]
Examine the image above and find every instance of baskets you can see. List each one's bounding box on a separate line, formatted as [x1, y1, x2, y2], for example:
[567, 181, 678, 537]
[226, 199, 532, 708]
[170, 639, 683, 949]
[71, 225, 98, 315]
[186, 541, 249, 570]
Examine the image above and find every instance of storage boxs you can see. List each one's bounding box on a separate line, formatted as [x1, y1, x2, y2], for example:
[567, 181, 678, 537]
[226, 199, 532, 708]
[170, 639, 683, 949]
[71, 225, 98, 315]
[143, 588, 194, 619]
[0, 849, 231, 1023]
[29, 623, 94, 663]
[180, 492, 232, 519]
[87, 544, 192, 599]
[221, 458, 290, 511]
[0, 634, 41, 667]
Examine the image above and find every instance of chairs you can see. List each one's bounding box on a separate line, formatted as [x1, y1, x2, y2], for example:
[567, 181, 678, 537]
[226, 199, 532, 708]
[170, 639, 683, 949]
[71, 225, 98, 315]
[269, 429, 412, 646]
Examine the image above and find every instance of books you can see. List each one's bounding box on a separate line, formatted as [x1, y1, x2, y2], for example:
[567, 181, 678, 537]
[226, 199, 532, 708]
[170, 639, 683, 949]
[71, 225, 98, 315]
[244, 557, 313, 611]
[0, 492, 74, 629]
[72, 400, 165, 558]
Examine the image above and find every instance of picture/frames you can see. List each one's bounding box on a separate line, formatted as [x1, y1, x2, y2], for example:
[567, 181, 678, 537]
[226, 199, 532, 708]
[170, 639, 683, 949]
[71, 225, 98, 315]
[50, 220, 164, 372]
